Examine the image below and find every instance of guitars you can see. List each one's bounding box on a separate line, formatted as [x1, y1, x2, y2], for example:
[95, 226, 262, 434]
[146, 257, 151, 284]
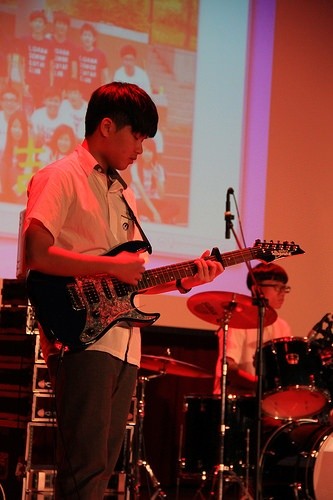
[24, 238, 306, 352]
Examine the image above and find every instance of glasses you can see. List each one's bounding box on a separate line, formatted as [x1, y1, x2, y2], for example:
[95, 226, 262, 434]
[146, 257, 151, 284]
[260, 284, 291, 293]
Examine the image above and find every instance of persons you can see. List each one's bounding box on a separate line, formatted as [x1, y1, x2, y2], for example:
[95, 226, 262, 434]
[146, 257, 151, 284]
[214, 262, 291, 395]
[23, 82, 223, 500]
[0, 11, 164, 224]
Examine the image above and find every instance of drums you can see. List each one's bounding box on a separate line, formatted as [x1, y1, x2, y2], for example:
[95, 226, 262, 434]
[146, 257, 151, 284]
[178, 393, 256, 478]
[257, 418, 333, 500]
[253, 337, 330, 420]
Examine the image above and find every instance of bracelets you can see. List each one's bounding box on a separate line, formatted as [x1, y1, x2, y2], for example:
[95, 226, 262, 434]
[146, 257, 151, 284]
[176, 279, 192, 294]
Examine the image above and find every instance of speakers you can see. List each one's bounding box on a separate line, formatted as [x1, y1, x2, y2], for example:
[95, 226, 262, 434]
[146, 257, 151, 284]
[177, 394, 259, 500]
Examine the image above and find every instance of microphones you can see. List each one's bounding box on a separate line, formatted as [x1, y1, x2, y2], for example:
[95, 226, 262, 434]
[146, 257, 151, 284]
[225, 187, 234, 239]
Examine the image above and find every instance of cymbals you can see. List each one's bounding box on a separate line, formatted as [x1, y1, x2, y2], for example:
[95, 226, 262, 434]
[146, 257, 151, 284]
[186, 291, 278, 330]
[139, 354, 214, 377]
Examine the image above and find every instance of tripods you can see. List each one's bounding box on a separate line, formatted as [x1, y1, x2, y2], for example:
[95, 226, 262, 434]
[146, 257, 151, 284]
[122, 372, 172, 500]
[194, 305, 254, 500]
